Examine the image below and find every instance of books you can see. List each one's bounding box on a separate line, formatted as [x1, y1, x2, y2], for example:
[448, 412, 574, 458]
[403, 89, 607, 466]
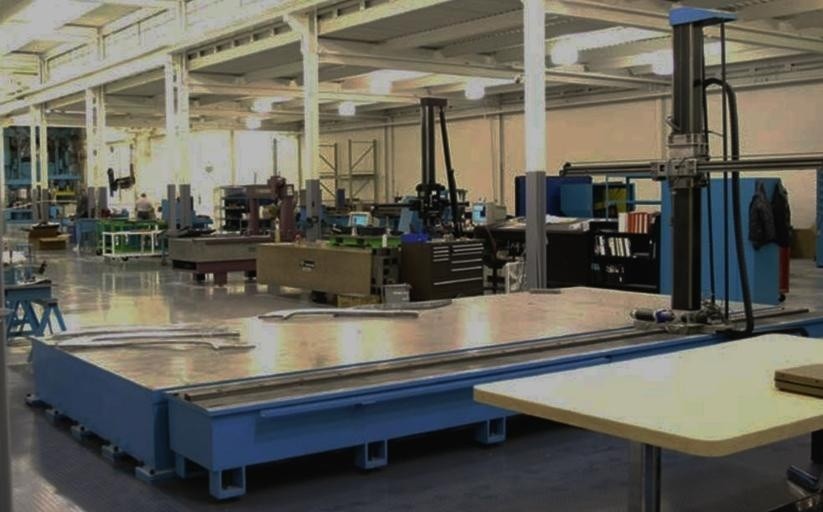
[595, 210, 650, 257]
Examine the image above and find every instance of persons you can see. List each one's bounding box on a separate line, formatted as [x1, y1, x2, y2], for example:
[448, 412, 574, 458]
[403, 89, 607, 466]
[156, 206, 163, 220]
[135, 193, 152, 220]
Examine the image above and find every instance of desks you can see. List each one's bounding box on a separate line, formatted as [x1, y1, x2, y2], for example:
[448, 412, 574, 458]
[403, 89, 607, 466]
[472, 332, 820, 511]
[399, 238, 484, 300]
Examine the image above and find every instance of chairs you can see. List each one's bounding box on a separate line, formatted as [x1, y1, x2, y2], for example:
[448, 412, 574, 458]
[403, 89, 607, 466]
[471, 224, 522, 294]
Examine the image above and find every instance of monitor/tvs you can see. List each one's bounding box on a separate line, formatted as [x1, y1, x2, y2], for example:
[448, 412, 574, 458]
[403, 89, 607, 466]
[347, 210, 382, 227]
[470, 201, 507, 225]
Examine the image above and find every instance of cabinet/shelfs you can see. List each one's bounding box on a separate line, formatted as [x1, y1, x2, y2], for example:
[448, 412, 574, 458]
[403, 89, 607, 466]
[219, 193, 277, 233]
[587, 214, 660, 295]
